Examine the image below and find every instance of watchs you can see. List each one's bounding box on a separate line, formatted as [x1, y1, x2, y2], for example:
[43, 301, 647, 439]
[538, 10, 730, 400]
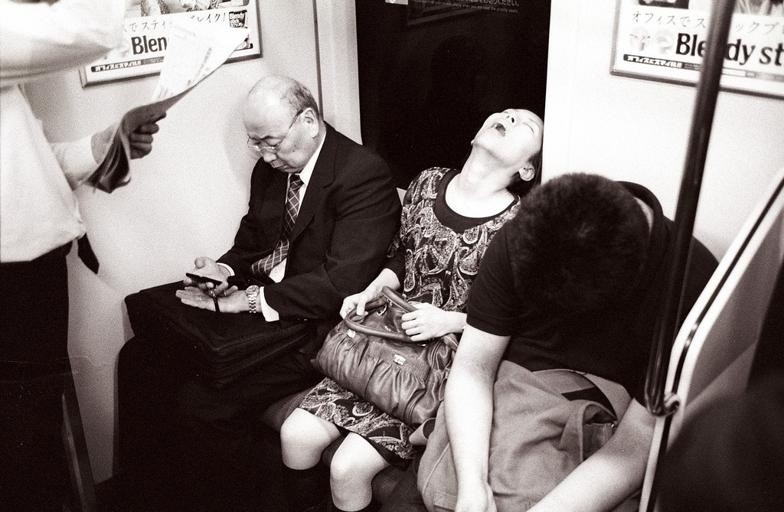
[245, 285, 260, 314]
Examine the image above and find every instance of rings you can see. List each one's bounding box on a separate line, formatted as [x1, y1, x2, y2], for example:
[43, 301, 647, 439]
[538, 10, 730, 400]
[211, 290, 216, 298]
[224, 289, 227, 297]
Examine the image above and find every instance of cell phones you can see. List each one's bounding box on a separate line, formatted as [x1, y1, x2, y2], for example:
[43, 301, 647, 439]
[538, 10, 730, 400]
[186, 272, 222, 286]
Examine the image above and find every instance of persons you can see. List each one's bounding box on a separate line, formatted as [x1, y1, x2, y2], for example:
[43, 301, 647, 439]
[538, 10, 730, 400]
[115, 78, 401, 512]
[418, 172, 721, 510]
[0, 0, 170, 512]
[277, 107, 543, 511]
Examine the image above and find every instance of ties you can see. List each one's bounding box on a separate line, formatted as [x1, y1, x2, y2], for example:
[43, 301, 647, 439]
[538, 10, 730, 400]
[250, 174, 305, 279]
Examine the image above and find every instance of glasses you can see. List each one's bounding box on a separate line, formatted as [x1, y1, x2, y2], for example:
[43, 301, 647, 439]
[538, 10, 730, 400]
[246, 108, 306, 156]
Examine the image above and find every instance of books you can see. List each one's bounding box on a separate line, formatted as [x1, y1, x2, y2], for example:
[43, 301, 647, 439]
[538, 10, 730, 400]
[77, 27, 250, 194]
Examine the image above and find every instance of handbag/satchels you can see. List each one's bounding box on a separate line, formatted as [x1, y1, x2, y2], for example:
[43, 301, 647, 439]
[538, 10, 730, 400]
[124, 273, 315, 383]
[415, 358, 631, 510]
[312, 284, 460, 428]
